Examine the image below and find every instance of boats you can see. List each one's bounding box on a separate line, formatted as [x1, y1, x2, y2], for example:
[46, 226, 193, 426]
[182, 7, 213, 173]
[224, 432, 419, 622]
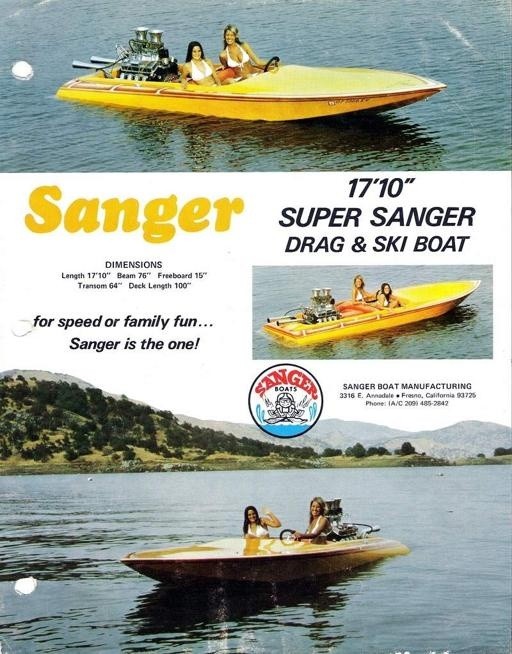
[122, 498, 410, 583]
[56, 26, 446, 122]
[259, 279, 480, 349]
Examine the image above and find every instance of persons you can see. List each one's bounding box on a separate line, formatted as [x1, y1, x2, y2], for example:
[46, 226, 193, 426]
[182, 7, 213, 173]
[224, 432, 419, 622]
[180, 40, 222, 94]
[351, 274, 372, 304]
[216, 24, 272, 79]
[291, 496, 329, 545]
[240, 506, 282, 539]
[379, 283, 405, 311]
